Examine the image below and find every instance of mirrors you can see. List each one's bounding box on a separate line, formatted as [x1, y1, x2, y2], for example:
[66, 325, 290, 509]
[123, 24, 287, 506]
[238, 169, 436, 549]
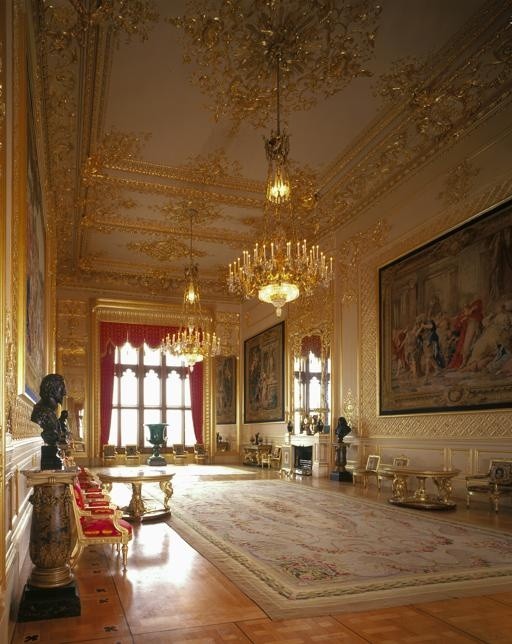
[55, 340, 90, 460]
[290, 328, 333, 437]
[210, 353, 239, 457]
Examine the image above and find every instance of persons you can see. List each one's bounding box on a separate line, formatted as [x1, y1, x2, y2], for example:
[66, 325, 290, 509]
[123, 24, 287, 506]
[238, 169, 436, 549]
[31, 373, 66, 446]
[391, 299, 512, 383]
[59, 410, 68, 432]
[334, 417, 352, 436]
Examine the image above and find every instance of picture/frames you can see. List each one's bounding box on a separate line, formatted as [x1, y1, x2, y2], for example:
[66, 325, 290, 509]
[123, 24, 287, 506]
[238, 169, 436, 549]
[214, 355, 237, 425]
[373, 195, 511, 421]
[14, 48, 51, 408]
[243, 319, 287, 426]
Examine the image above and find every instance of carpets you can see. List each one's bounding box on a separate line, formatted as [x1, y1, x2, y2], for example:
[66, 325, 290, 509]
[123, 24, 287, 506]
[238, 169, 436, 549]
[131, 477, 512, 625]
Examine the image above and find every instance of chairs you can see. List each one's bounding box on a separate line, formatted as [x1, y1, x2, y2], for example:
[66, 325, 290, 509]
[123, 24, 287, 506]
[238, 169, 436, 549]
[378, 456, 410, 490]
[465, 458, 511, 514]
[192, 442, 211, 466]
[171, 442, 190, 466]
[261, 445, 283, 470]
[100, 444, 120, 465]
[67, 463, 133, 577]
[351, 453, 382, 492]
[124, 444, 141, 465]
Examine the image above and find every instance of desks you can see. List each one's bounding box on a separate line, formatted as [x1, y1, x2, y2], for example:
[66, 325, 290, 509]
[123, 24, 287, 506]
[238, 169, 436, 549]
[384, 461, 460, 511]
[96, 465, 178, 526]
[242, 444, 272, 467]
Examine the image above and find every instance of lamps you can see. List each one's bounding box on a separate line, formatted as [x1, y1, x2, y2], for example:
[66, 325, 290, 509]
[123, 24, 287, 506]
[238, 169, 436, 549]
[222, 39, 337, 316]
[159, 207, 223, 374]
